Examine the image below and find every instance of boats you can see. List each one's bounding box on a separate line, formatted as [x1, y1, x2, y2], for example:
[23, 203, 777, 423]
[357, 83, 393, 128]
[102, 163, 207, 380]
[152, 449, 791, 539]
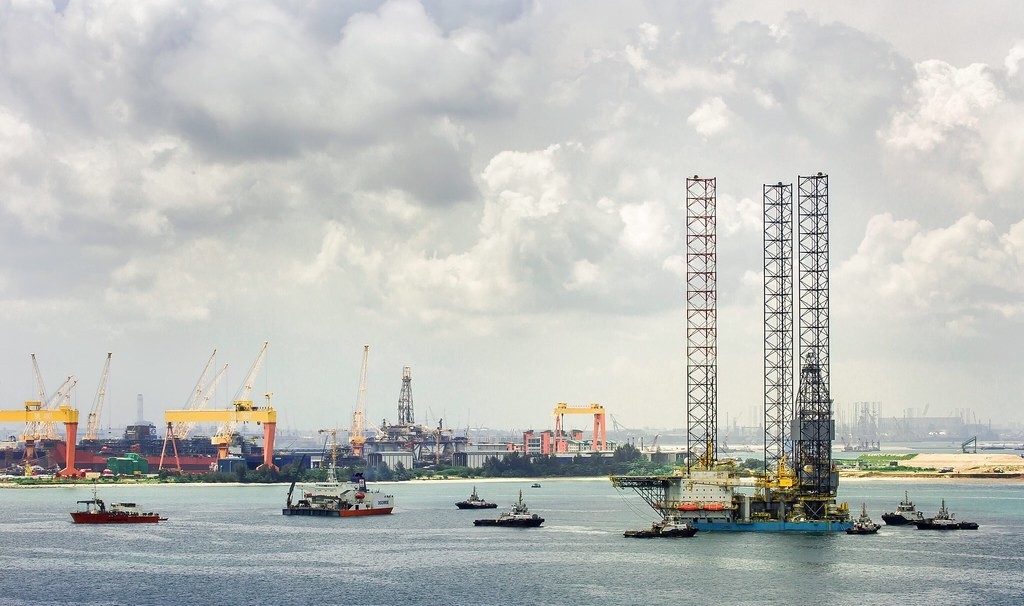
[845, 503, 881, 535]
[283, 453, 394, 517]
[916, 498, 979, 530]
[69, 487, 169, 525]
[473, 490, 545, 528]
[841, 437, 881, 451]
[881, 490, 924, 525]
[623, 515, 699, 538]
[455, 485, 498, 509]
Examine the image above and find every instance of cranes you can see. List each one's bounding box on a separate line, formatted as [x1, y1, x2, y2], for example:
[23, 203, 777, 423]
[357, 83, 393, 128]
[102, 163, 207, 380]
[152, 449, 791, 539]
[81, 352, 114, 444]
[167, 348, 229, 441]
[211, 341, 268, 445]
[17, 352, 78, 443]
[349, 344, 371, 445]
